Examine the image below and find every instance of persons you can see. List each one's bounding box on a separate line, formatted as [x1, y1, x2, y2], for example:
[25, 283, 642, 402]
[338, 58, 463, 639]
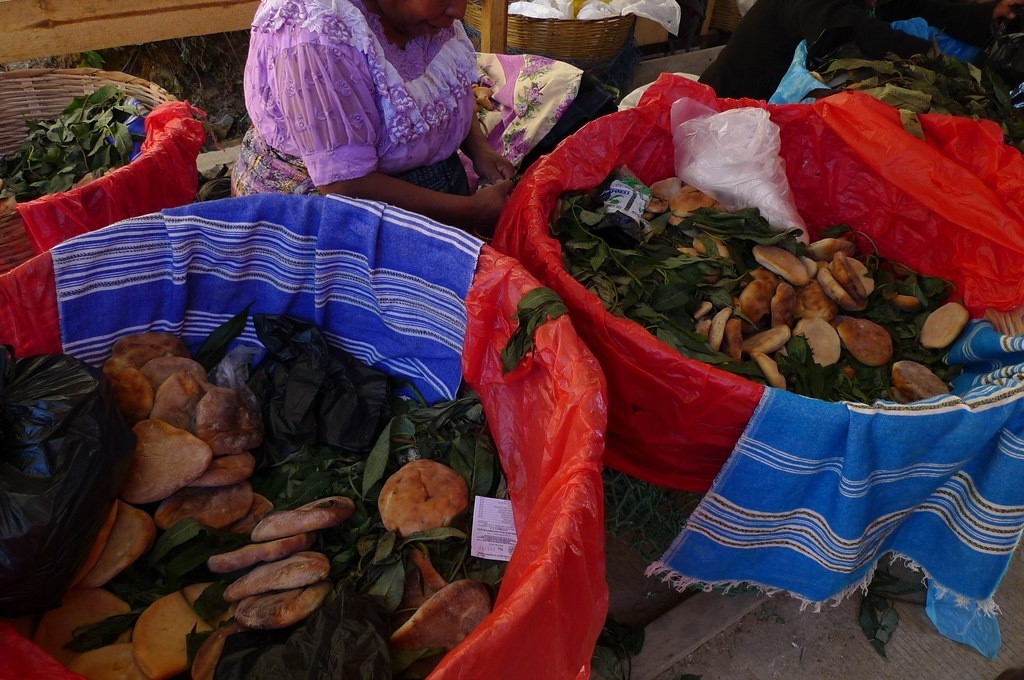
[231, 0, 514, 247]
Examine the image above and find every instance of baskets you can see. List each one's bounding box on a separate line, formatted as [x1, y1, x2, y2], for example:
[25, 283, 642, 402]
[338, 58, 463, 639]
[0, 68, 178, 274]
[709, 0, 748, 35]
[463, 0, 636, 76]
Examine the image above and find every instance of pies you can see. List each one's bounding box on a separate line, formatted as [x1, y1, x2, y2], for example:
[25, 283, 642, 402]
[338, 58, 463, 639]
[29, 330, 491, 680]
[0, 168, 121, 215]
[647, 176, 969, 399]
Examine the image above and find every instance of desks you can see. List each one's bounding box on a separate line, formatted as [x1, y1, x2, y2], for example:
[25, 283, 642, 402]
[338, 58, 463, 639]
[0, 0, 510, 69]
